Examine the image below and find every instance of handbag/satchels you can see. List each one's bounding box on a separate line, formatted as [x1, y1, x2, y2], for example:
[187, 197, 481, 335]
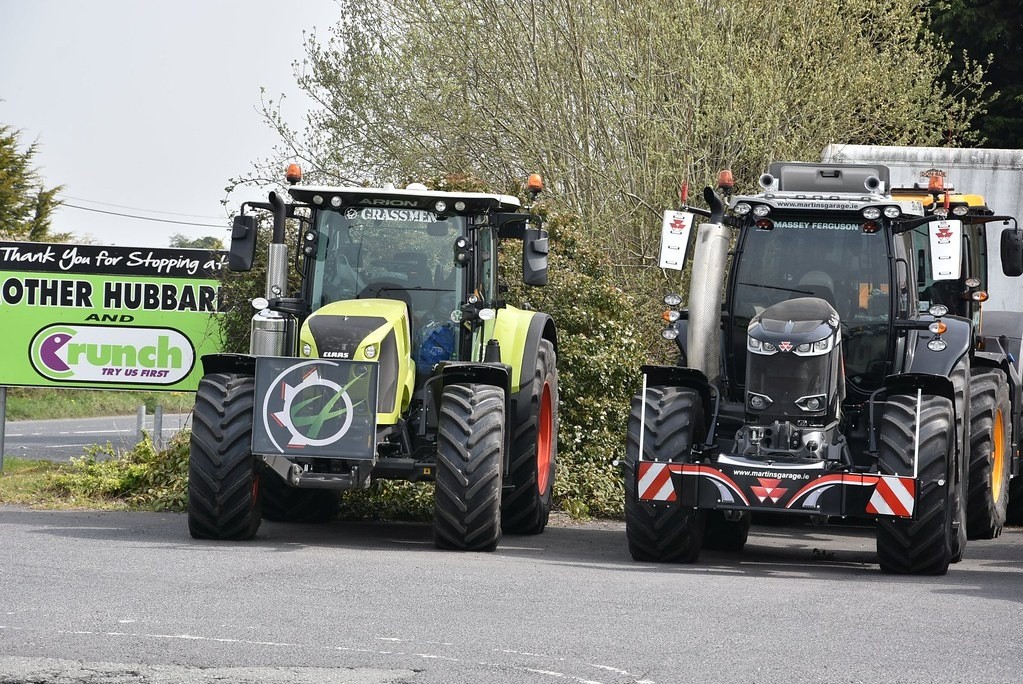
[412, 322, 455, 375]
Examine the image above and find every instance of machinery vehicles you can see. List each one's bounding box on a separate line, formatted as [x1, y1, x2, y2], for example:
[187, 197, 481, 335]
[619, 147, 1023, 588]
[190, 163, 564, 555]
